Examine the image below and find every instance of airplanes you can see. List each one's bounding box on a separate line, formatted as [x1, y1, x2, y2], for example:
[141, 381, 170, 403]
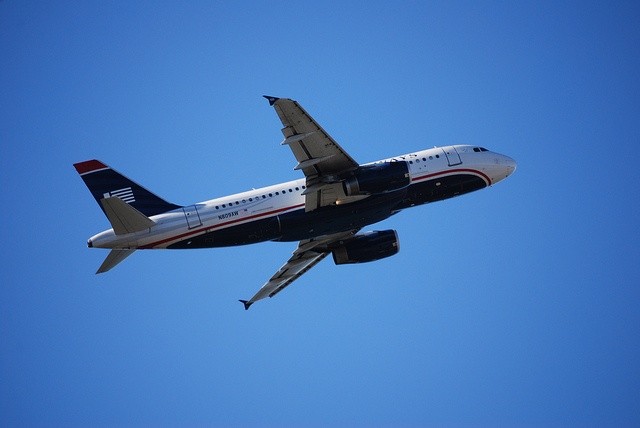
[72, 94, 518, 310]
